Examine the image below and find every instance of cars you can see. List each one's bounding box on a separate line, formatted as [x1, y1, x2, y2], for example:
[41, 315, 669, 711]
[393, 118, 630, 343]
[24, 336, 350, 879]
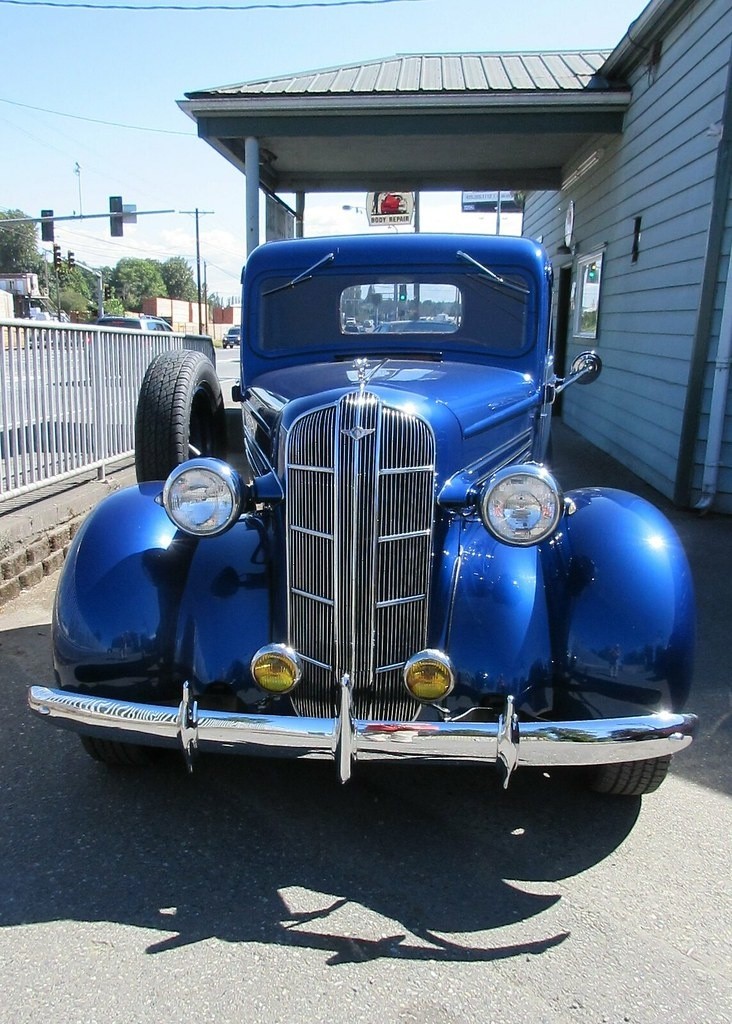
[21, 232, 692, 796]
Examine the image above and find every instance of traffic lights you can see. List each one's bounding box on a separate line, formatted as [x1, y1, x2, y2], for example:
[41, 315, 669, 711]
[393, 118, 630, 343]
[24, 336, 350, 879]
[67, 249, 75, 268]
[52, 244, 63, 270]
